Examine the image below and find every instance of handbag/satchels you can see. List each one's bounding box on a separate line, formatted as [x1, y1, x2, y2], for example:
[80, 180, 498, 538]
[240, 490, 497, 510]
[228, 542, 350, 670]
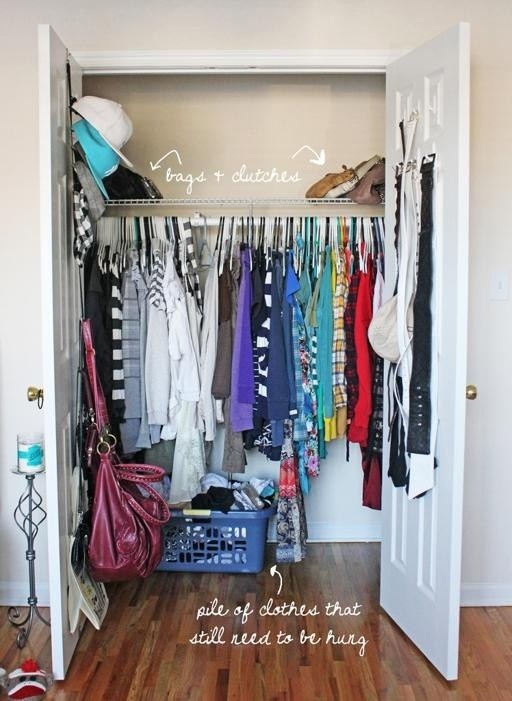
[90, 431, 171, 582]
[348, 159, 386, 207]
[78, 426, 101, 557]
[304, 166, 354, 200]
[325, 153, 380, 198]
[102, 164, 163, 203]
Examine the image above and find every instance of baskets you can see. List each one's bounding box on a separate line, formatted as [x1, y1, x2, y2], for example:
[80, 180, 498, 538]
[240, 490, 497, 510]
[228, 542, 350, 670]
[140, 488, 282, 577]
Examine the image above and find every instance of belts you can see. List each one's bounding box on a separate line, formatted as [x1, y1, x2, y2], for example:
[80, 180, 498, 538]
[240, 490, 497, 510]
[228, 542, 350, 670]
[405, 152, 439, 457]
[395, 107, 419, 436]
[384, 158, 417, 493]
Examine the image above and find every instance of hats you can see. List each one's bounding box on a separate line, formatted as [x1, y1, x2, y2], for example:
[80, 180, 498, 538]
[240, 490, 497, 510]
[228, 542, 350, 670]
[71, 93, 137, 170]
[73, 162, 108, 223]
[67, 114, 122, 202]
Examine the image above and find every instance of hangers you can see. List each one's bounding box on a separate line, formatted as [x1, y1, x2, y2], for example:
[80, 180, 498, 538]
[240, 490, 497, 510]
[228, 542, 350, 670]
[91, 213, 387, 279]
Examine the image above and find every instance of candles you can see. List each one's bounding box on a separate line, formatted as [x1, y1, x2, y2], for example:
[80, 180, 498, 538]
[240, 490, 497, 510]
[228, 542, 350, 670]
[15, 418, 46, 474]
